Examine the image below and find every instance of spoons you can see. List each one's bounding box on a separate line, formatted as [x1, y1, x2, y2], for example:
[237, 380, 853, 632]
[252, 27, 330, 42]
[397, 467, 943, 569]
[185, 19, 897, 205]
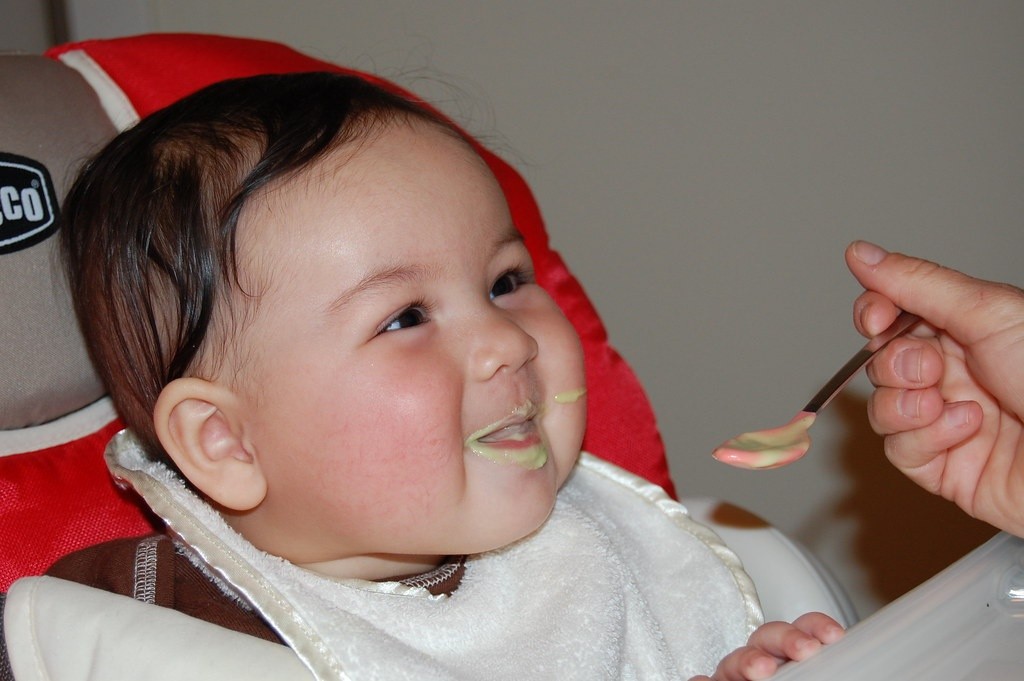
[712, 311, 923, 468]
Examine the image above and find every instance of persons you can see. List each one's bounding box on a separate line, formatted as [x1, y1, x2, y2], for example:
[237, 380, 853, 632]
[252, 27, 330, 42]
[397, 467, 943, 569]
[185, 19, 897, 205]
[0, 72, 852, 681]
[845, 240, 1023, 546]
